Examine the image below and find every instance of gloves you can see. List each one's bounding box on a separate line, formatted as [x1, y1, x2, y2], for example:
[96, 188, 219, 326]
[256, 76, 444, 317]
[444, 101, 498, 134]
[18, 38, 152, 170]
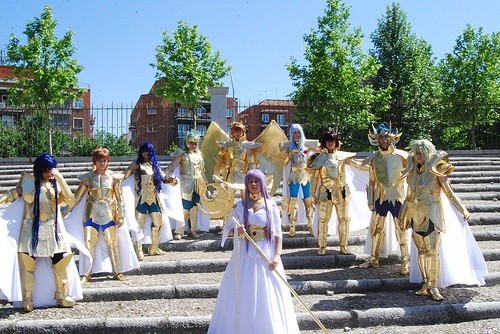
[399, 197, 412, 231]
[349, 159, 369, 172]
[310, 178, 318, 205]
[441, 181, 470, 221]
[368, 185, 376, 212]
[50, 168, 72, 197]
[116, 218, 124, 228]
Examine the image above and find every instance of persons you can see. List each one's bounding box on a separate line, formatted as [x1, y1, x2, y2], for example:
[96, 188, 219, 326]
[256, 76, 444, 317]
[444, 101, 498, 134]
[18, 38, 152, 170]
[207, 170, 300, 334]
[397, 140, 489, 301]
[282, 124, 320, 237]
[122, 142, 178, 261]
[69, 149, 126, 284]
[212, 122, 263, 206]
[359, 120, 411, 274]
[165, 132, 207, 240]
[309, 131, 370, 256]
[0, 154, 77, 311]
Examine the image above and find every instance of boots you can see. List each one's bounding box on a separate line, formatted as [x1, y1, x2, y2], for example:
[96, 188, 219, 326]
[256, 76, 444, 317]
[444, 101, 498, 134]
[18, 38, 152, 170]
[134, 210, 147, 261]
[318, 200, 332, 255]
[359, 212, 386, 269]
[335, 199, 350, 255]
[18, 252, 36, 311]
[174, 209, 190, 240]
[187, 206, 200, 238]
[412, 232, 429, 295]
[393, 217, 410, 274]
[148, 212, 165, 256]
[302, 196, 315, 236]
[103, 226, 126, 281]
[81, 226, 99, 284]
[288, 197, 299, 236]
[424, 231, 444, 301]
[52, 252, 76, 307]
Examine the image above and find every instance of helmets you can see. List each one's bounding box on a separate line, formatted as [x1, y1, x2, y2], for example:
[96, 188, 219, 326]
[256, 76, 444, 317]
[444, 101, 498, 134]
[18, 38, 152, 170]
[230, 122, 246, 142]
[185, 132, 198, 149]
